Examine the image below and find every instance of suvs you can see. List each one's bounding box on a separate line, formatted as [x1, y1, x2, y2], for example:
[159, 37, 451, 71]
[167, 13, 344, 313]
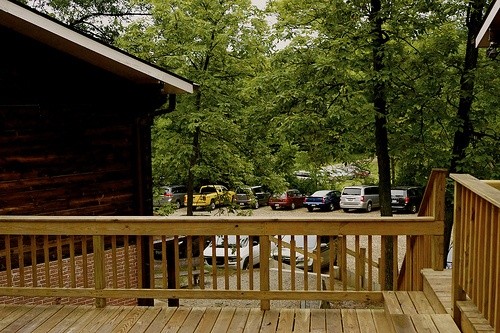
[233, 185, 272, 209]
[389, 186, 424, 214]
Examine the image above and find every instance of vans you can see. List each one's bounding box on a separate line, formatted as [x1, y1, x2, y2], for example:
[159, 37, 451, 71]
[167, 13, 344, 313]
[159, 185, 197, 209]
[339, 185, 380, 212]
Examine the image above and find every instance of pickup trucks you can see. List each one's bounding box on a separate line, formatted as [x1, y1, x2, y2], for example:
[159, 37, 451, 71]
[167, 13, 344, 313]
[184, 184, 237, 210]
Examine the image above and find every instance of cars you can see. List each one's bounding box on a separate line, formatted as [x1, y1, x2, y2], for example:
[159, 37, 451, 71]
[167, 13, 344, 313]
[153, 235, 211, 261]
[292, 164, 370, 180]
[202, 236, 277, 272]
[268, 188, 308, 210]
[302, 189, 342, 212]
[271, 234, 338, 272]
